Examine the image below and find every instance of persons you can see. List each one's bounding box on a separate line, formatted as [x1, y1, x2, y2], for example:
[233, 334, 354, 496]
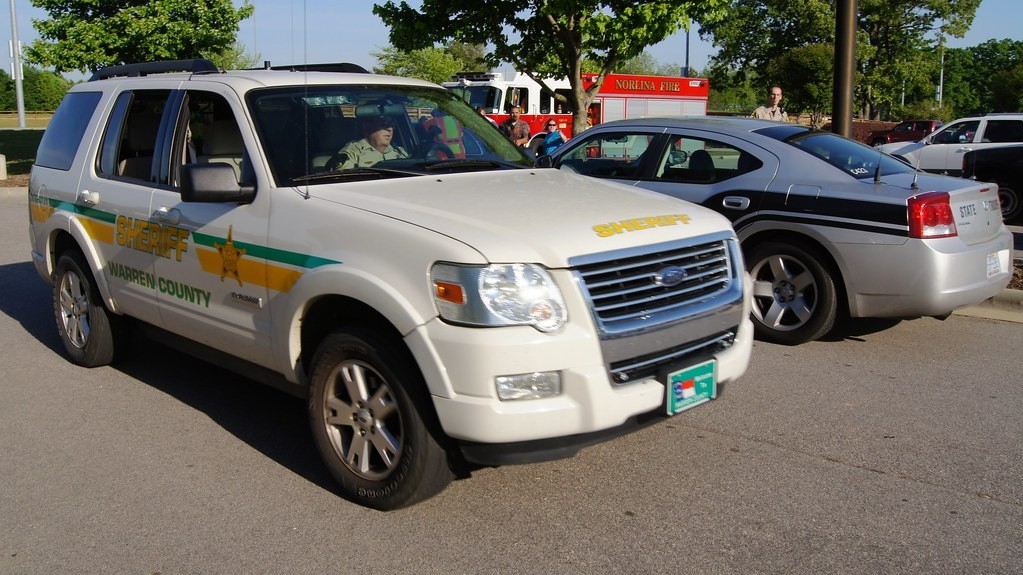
[476, 107, 499, 129]
[542, 118, 567, 159]
[749, 86, 789, 122]
[496, 106, 531, 147]
[422, 107, 466, 161]
[329, 105, 408, 172]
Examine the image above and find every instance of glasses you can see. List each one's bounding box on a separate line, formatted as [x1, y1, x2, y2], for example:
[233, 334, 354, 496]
[547, 123, 557, 126]
[378, 119, 398, 128]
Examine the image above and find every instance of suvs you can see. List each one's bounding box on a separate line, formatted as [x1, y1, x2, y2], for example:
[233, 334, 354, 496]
[873, 111, 1023, 176]
[866, 119, 944, 147]
[28, 57, 755, 511]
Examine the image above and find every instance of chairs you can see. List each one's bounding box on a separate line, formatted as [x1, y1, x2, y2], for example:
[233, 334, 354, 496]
[737, 153, 749, 173]
[119, 113, 162, 182]
[310, 117, 355, 174]
[197, 121, 242, 183]
[687, 149, 715, 183]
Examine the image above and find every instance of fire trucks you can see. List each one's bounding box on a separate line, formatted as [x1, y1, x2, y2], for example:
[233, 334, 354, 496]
[441, 70, 710, 166]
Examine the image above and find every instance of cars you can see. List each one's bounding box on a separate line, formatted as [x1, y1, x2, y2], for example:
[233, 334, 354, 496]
[410, 119, 506, 163]
[547, 114, 1016, 346]
[961, 146, 1023, 220]
